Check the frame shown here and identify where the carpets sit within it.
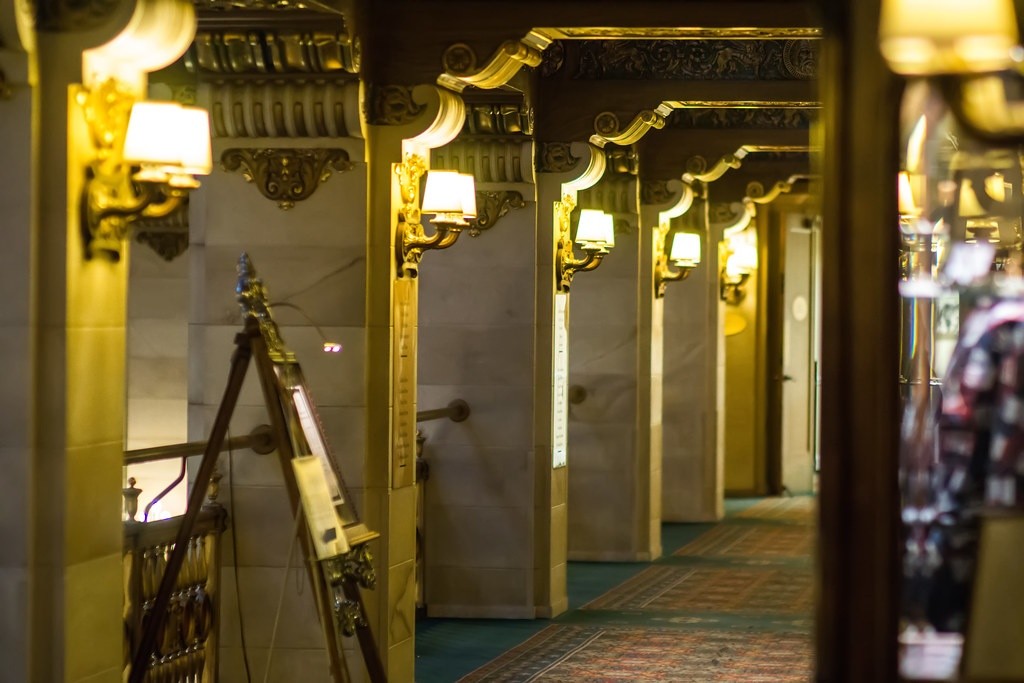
[459,494,814,683]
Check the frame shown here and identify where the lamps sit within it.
[654,232,701,298]
[83,98,213,263]
[556,208,615,292]
[720,246,758,300]
[396,168,478,277]
